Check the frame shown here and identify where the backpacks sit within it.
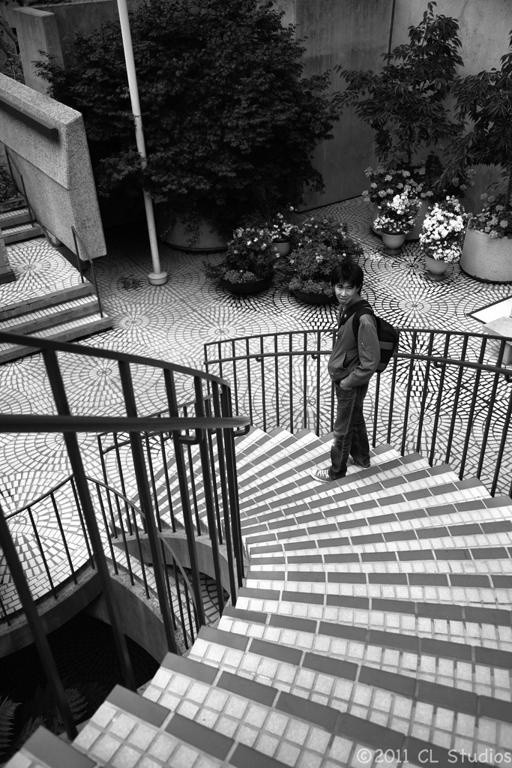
[353,308,397,373]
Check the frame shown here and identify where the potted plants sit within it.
[30,0,340,257]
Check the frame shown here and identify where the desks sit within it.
[465,293,512,372]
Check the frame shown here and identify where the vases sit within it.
[421,253,449,282]
[460,227,512,283]
[381,229,406,255]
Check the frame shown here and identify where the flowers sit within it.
[362,163,423,236]
[453,164,512,241]
[418,195,467,262]
[201,204,367,308]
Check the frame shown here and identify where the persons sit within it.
[310,261,380,483]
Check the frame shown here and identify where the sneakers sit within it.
[348,454,371,469]
[309,469,333,483]
